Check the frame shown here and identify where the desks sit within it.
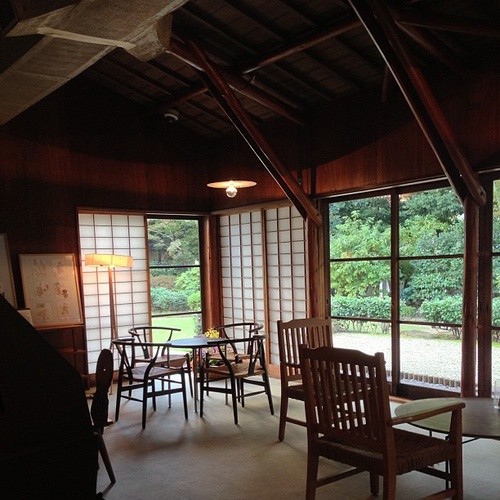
[165,336,230,400]
[395,396,500,444]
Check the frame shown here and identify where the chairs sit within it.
[90,349,117,483]
[111,338,188,430]
[298,344,467,500]
[198,333,274,424]
[127,326,194,397]
[276,315,371,442]
[202,322,264,402]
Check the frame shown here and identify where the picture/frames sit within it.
[17,252,85,331]
[1,232,17,310]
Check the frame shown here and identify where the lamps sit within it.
[85,254,135,395]
[205,129,258,199]
